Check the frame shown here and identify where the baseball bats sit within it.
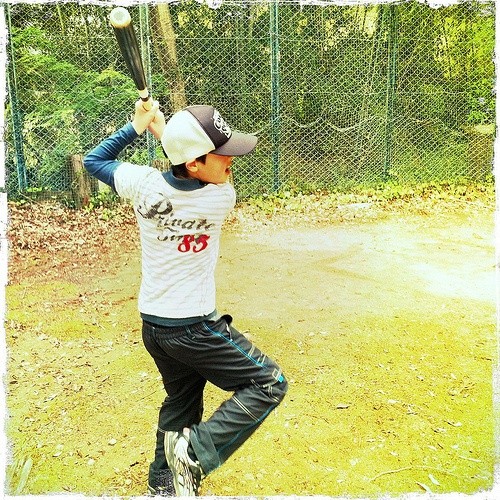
[110,8,153,111]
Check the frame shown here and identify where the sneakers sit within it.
[148,484,175,496]
[164,427,207,497]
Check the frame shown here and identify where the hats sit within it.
[160,105,258,166]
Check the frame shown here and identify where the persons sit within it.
[83,97,288,497]
